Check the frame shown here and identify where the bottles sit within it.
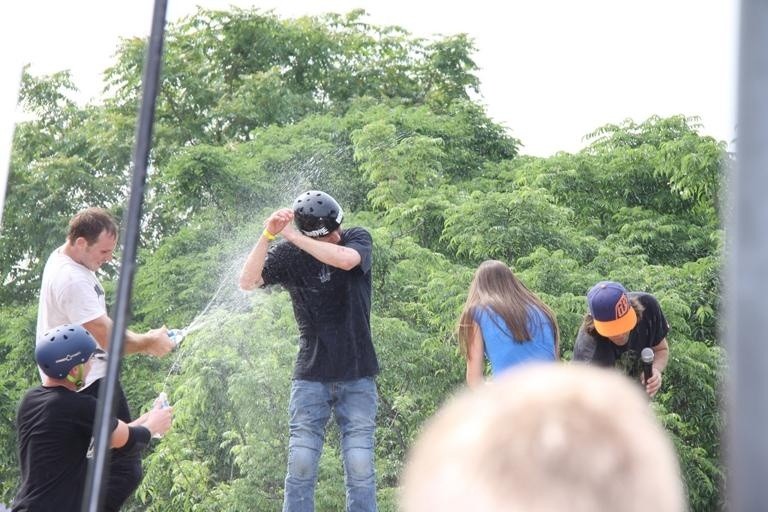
[168,326,190,345]
[150,389,171,438]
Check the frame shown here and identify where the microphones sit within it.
[641,347,654,384]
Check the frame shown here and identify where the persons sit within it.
[569,280,672,400]
[393,361,688,512]
[11,324,176,512]
[240,188,379,511]
[455,258,560,388]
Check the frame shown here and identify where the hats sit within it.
[589,280,640,339]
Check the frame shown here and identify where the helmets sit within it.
[293,190,346,238]
[33,323,104,381]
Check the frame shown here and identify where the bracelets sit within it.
[29,207,176,423]
[126,424,152,453]
[262,226,281,242]
